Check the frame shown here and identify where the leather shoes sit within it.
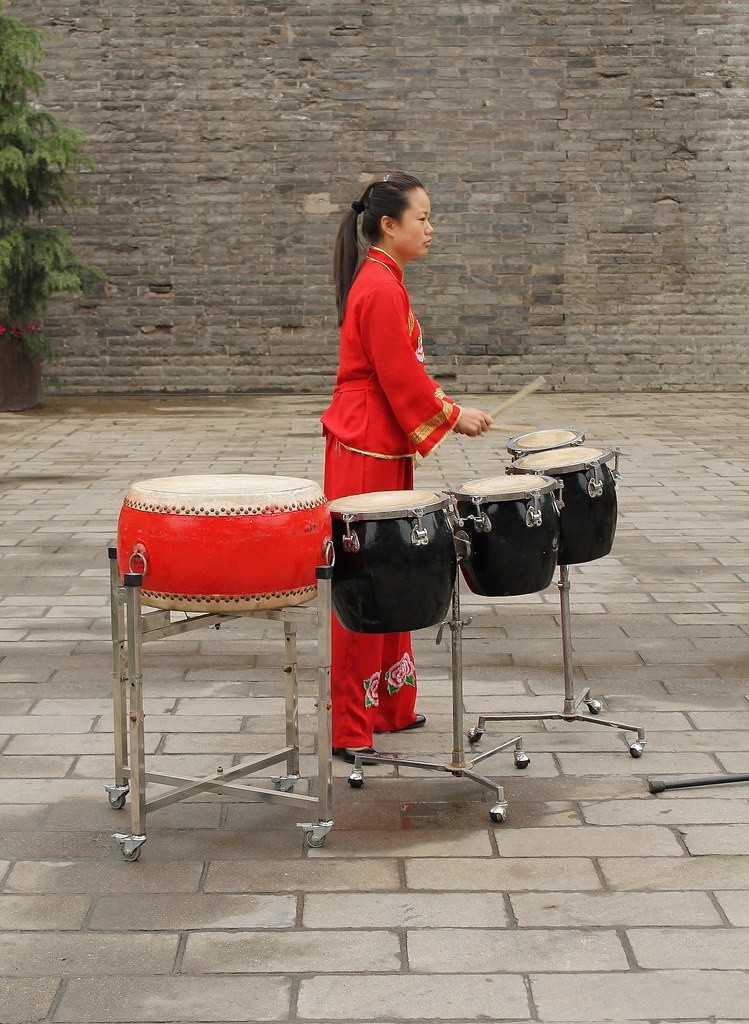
[408,714,427,730]
[331,745,380,765]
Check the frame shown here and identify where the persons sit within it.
[320,172,493,765]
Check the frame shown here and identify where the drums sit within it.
[450,473,560,598]
[328,488,458,635]
[506,426,585,464]
[508,442,617,566]
[116,472,334,614]
